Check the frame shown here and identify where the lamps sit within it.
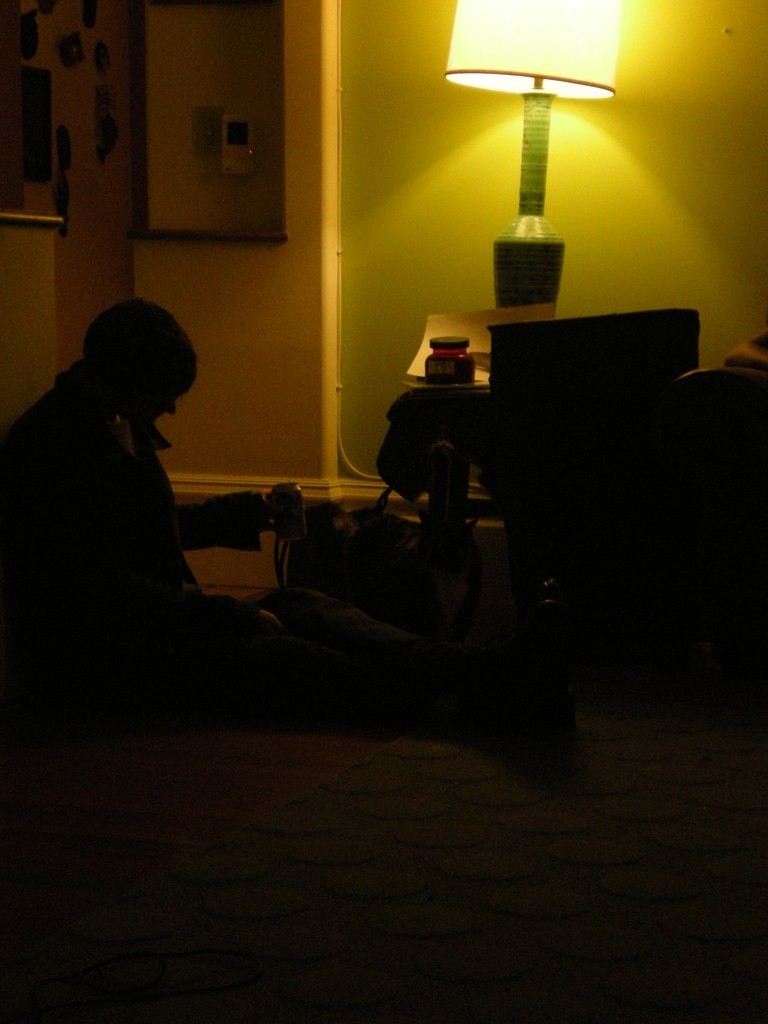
[444,1,623,305]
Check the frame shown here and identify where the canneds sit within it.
[424,337,475,386]
[271,482,307,540]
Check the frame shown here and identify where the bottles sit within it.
[425,337,474,383]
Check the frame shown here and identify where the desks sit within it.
[379,388,494,541]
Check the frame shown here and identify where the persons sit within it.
[1,300,579,751]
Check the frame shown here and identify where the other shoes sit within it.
[509,601,578,750]
